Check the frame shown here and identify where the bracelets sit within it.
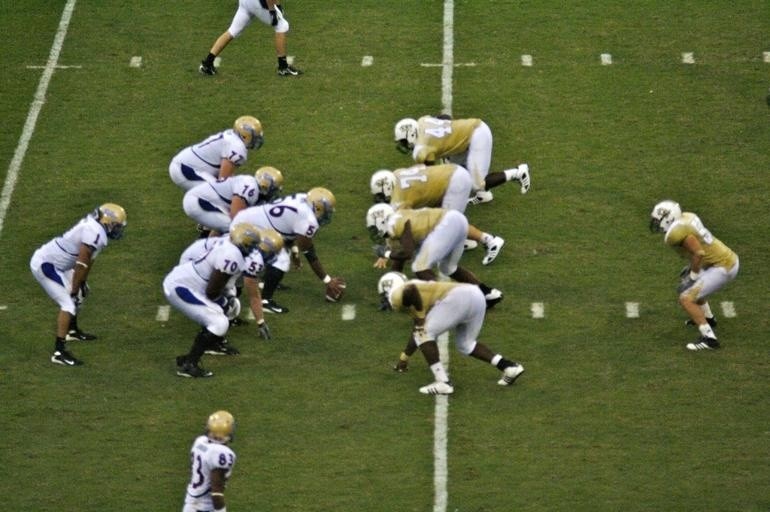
[385,250,392,259]
[399,352,410,363]
[689,268,700,281]
[214,505,226,512]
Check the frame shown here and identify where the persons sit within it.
[179,409,237,512]
[170,115,263,236]
[370,163,506,266]
[649,199,740,349]
[374,270,525,394]
[30,202,128,365]
[182,167,283,238]
[163,223,284,376]
[229,187,346,314]
[392,114,530,194]
[366,203,502,310]
[197,0,304,76]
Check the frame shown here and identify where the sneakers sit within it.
[199,64,217,75]
[685,315,718,327]
[485,288,504,309]
[65,330,96,342]
[482,236,505,265]
[686,337,720,351]
[258,282,289,314]
[176,317,250,378]
[467,190,493,205]
[51,351,84,366]
[515,163,531,194]
[464,239,479,250]
[497,363,525,385]
[277,65,303,76]
[418,382,456,395]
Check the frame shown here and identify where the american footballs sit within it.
[326,277,346,302]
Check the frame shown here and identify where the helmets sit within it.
[378,271,408,311]
[230,223,284,260]
[304,187,337,226]
[365,202,395,246]
[94,203,128,240]
[207,410,235,443]
[254,166,284,195]
[394,118,420,154]
[370,169,397,203]
[234,115,264,151]
[650,200,682,234]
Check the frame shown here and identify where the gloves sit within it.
[270,5,282,26]
[71,280,88,306]
[677,266,696,294]
[257,323,273,340]
